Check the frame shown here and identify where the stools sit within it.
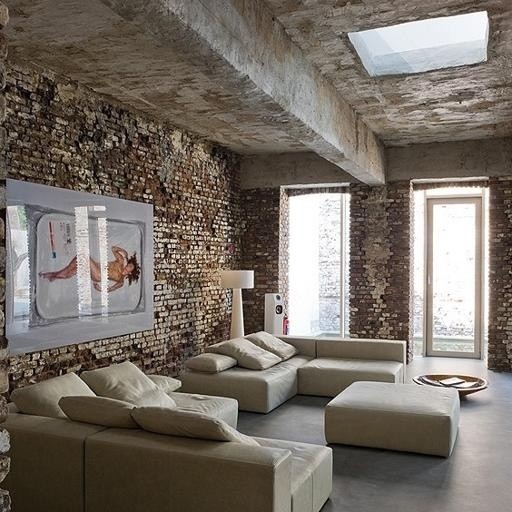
[324,381,460,456]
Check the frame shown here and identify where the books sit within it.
[438,376,464,387]
[421,376,443,386]
[451,381,478,388]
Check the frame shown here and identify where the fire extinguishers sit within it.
[283,314,289,335]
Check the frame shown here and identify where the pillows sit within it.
[79,361,177,410]
[146,375,182,395]
[10,372,98,419]
[132,406,262,448]
[183,353,237,373]
[217,337,282,370]
[244,330,300,362]
[58,396,141,430]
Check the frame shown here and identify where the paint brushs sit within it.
[49,221,56,258]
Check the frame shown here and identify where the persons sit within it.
[37,243,142,294]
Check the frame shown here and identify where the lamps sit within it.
[221,270,254,340]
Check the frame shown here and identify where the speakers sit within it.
[264,292,284,335]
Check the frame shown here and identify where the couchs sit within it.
[182,335,407,414]
[0,374,333,512]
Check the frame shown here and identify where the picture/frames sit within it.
[5,178,155,357]
